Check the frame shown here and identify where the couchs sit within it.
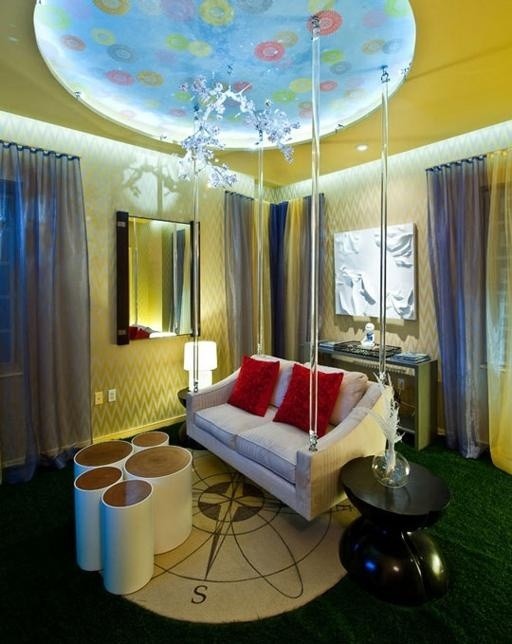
[187,352,392,521]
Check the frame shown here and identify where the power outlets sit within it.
[108,389,116,402]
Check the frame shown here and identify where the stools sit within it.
[67,429,193,594]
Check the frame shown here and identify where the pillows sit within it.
[226,353,344,436]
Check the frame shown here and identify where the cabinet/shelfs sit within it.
[329,350,438,451]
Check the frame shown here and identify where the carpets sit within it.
[99,449,359,625]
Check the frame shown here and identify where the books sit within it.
[388,351,430,364]
[319,341,338,351]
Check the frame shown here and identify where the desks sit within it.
[337,453,451,606]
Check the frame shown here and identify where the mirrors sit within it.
[124,212,194,343]
